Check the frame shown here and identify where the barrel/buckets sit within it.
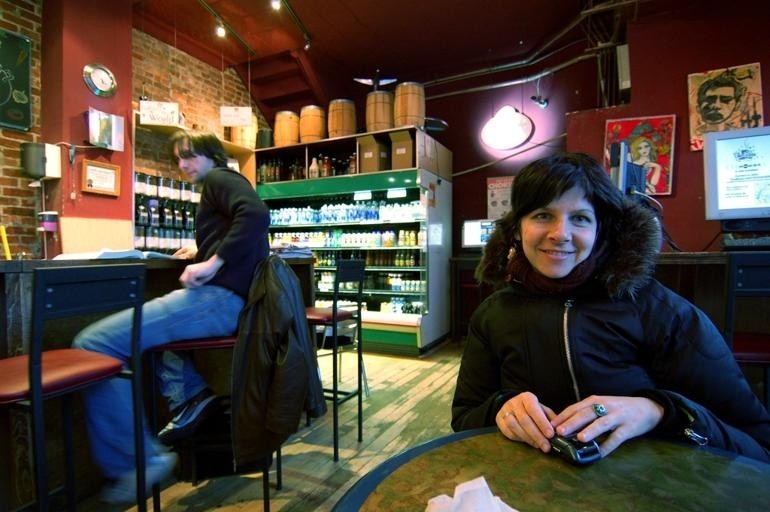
[231,113,259,148]
[274,81,425,148]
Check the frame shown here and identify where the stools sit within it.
[731,330,769,408]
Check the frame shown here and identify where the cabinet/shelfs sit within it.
[131,108,257,252]
[253,125,454,360]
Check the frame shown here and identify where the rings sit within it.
[590,401,608,417]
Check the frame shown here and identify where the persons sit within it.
[604,122,670,193]
[67,126,270,507]
[450,150,768,466]
[694,71,751,131]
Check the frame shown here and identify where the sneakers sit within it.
[157,387,219,441]
[100,452,179,505]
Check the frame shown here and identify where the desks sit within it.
[725,250,770,351]
[652,250,732,338]
[327,424,770,511]
[450,253,488,341]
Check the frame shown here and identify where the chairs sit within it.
[149,255,283,511]
[305,257,366,463]
[0,262,147,512]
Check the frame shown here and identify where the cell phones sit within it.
[550,433,601,465]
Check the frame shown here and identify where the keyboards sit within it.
[721,237,770,250]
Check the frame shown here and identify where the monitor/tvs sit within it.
[609,141,647,204]
[460,218,500,251]
[703,124,769,220]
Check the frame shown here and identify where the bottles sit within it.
[256,151,357,183]
[136,171,199,255]
[268,201,426,315]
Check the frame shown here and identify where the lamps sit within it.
[480,86,534,151]
